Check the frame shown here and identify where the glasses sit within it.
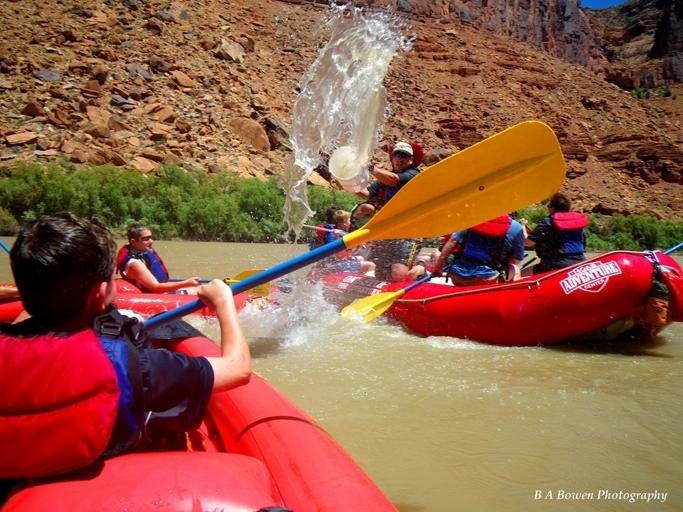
[546,204,554,209]
[139,236,153,241]
[395,152,410,158]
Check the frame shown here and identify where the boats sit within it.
[1,312,402,510]
[305,244,683,355]
[0,280,274,321]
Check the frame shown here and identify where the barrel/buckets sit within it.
[327,145,370,193]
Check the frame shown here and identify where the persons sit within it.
[326,140,421,279]
[426,210,529,288]
[117,224,201,293]
[518,191,588,275]
[308,204,450,301]
[0,209,253,496]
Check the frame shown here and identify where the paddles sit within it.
[340,266,451,322]
[166,269,269,293]
[143,120,563,329]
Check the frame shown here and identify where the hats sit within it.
[393,142,414,156]
